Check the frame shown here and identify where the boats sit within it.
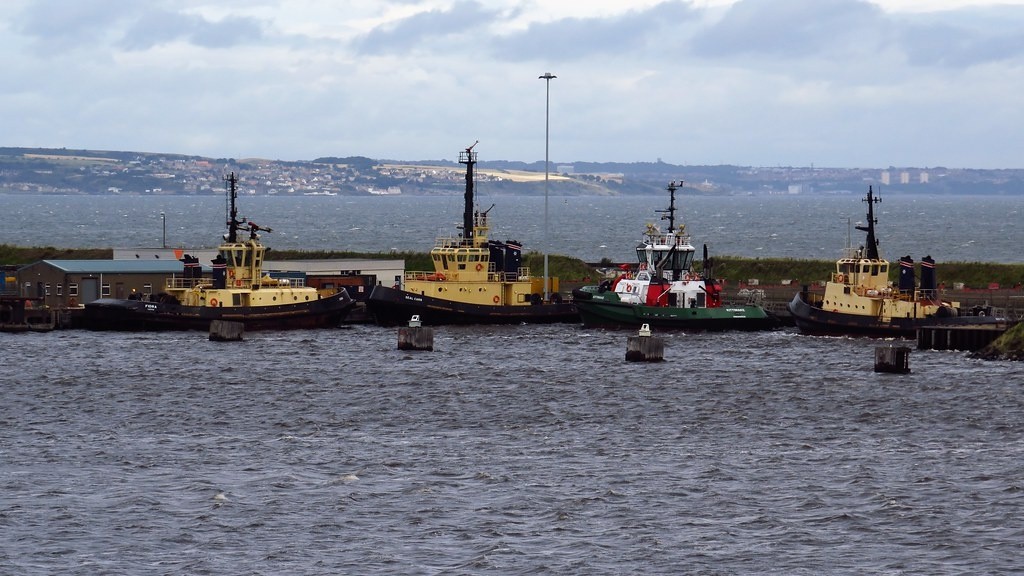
[786,182,1017,348]
[360,139,575,322]
[88,171,360,331]
[570,176,777,332]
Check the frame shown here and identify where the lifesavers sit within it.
[837,275,843,282]
[979,311,986,316]
[627,285,631,292]
[493,296,500,303]
[476,264,482,271]
[210,298,218,306]
[685,273,691,280]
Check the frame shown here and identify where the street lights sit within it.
[160,211,166,249]
[537,73,557,306]
